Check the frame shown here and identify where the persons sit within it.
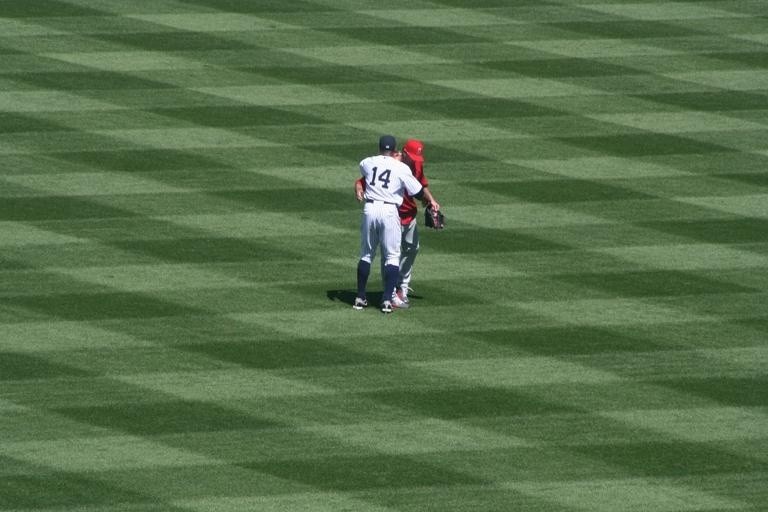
[353,136,440,313]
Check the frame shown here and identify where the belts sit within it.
[365,198,396,205]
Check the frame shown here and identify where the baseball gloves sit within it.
[424,204,444,229]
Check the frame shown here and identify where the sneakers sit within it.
[352,295,368,310]
[380,285,414,312]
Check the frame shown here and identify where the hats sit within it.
[403,140,425,162]
[379,135,396,151]
